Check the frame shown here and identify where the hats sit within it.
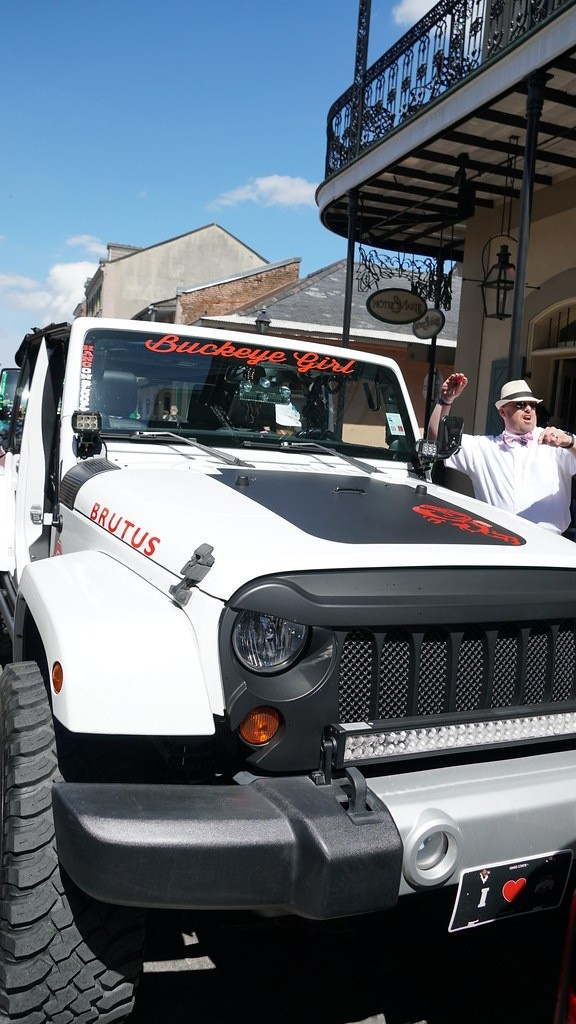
[494,379,543,411]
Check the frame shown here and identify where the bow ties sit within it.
[502,426,536,447]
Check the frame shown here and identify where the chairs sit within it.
[93,362,307,437]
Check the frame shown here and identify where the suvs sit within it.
[0,312,576,1024]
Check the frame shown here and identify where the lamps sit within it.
[476,134,520,322]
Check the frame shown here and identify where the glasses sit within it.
[504,401,537,409]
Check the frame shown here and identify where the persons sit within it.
[162,405,185,422]
[426,373,575,541]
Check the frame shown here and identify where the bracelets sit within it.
[436,397,454,406]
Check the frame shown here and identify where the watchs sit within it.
[559,431,575,449]
